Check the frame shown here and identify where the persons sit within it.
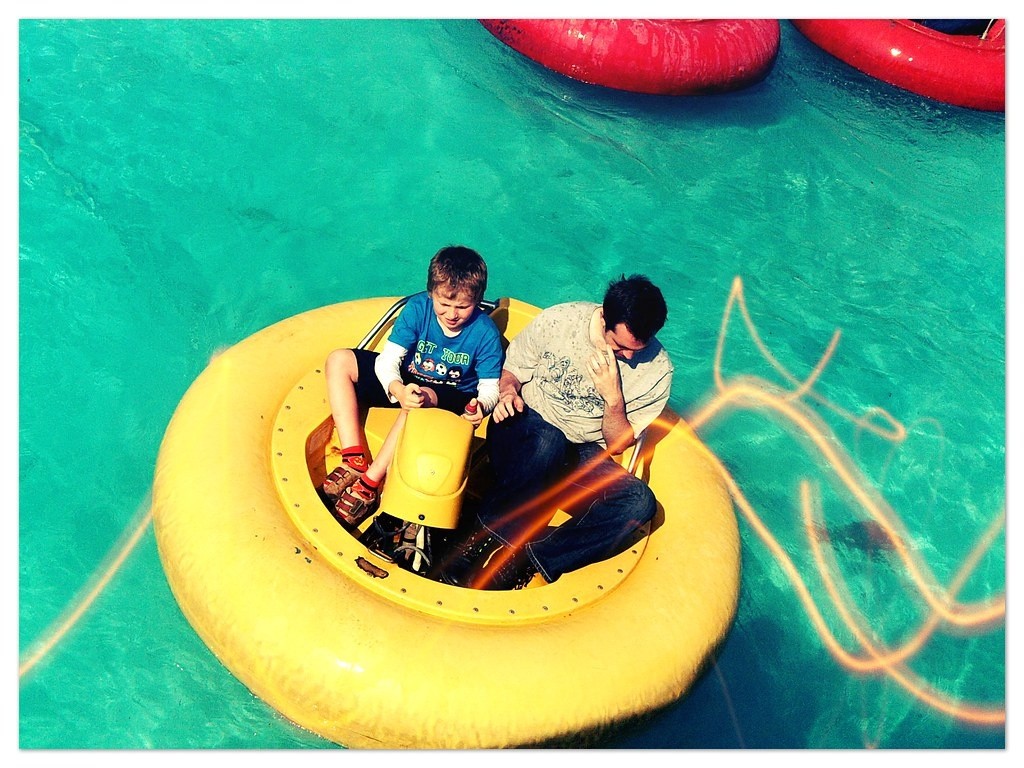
[316,246,504,528]
[435,273,675,592]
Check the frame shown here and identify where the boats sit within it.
[477,18,781,93]
[793,19,1005,113]
[151,294,740,751]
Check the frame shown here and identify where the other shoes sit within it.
[466,543,537,591]
[434,516,500,587]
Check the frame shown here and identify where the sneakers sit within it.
[330,478,377,531]
[316,464,366,506]
